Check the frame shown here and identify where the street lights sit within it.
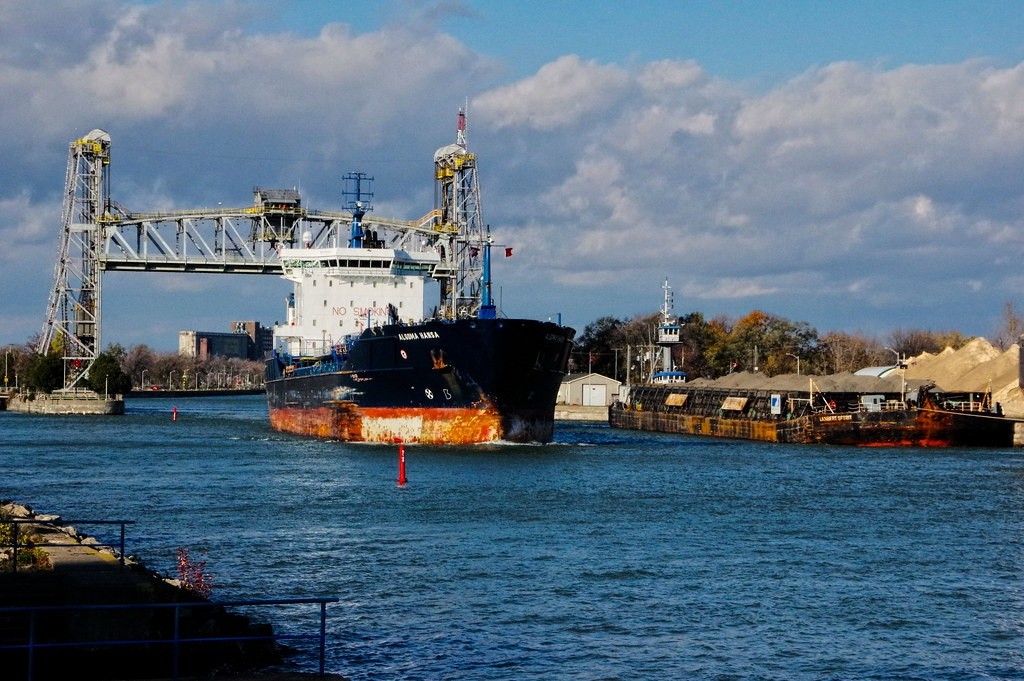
[195,372,202,390]
[785,352,800,375]
[4,351,9,392]
[141,369,148,390]
[207,372,258,389]
[105,374,109,400]
[169,369,176,390]
[884,347,899,368]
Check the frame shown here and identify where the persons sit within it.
[828,398,836,413]
[346,336,353,351]
[278,346,281,356]
[330,346,338,361]
[837,399,848,413]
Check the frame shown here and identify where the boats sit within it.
[259,163,576,452]
[608,381,1014,450]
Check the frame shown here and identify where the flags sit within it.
[730,363,740,369]
[505,248,512,258]
[50,326,63,352]
[590,356,598,362]
